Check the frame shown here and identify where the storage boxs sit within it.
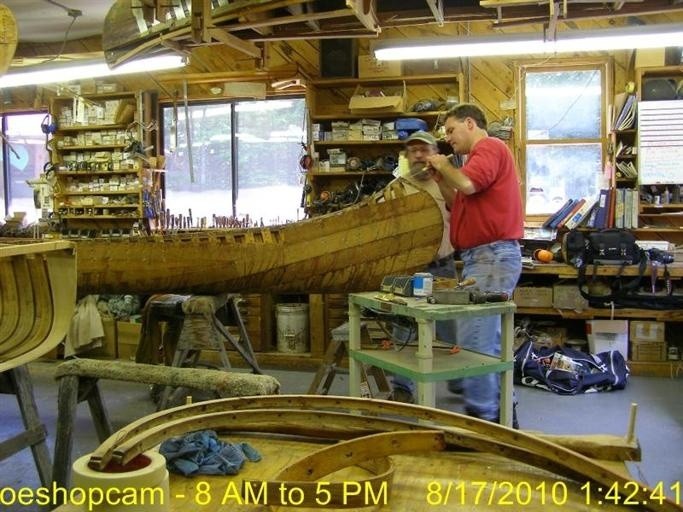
[358,55,402,76]
[513,279,668,361]
[58,316,142,360]
[348,96,403,113]
[634,47,665,68]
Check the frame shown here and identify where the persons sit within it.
[426,103,524,429]
[374,131,463,406]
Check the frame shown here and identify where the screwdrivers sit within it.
[453,276,477,289]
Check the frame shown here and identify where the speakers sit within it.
[319,38,359,79]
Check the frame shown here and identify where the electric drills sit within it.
[469,287,508,304]
[532,248,562,264]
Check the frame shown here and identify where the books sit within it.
[542,187,639,233]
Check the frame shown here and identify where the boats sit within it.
[1,175,445,296]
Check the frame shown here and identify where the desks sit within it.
[51,436,648,512]
[349,291,517,430]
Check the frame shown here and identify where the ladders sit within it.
[306,322,395,401]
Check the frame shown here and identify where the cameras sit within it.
[649,247,674,264]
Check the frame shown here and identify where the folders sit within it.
[594,189,609,230]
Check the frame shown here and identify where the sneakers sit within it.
[389,388,414,404]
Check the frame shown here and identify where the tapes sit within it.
[71,450,169,512]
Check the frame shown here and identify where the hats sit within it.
[407,131,436,145]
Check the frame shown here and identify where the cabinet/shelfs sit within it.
[610,65,683,232]
[50,88,144,236]
[512,264,683,378]
[224,293,271,351]
[306,72,466,357]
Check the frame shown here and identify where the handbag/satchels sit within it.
[562,228,641,265]
[514,339,629,395]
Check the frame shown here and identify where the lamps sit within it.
[0,54,191,88]
[374,3,683,60]
